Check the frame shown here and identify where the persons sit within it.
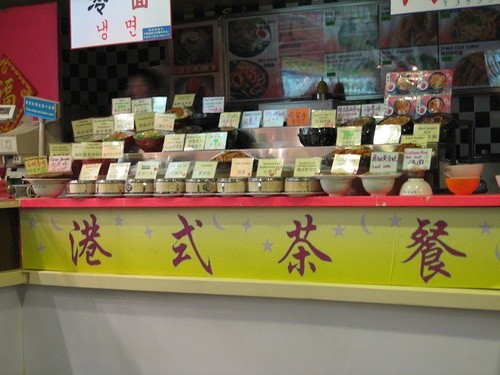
[129,68,164,99]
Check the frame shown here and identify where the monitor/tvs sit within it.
[1,118,61,157]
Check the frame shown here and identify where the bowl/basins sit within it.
[320,178,354,195]
[228,19,272,57]
[166,107,194,127]
[444,164,484,195]
[75,129,168,151]
[400,177,434,195]
[385,81,427,115]
[28,181,65,199]
[72,158,118,175]
[231,59,270,98]
[495,175,500,189]
[297,113,459,146]
[361,178,395,196]
[393,73,447,114]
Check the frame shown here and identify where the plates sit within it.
[314,175,357,179]
[21,179,72,180]
[358,173,402,178]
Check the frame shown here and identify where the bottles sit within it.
[335,77,344,94]
[317,77,328,94]
